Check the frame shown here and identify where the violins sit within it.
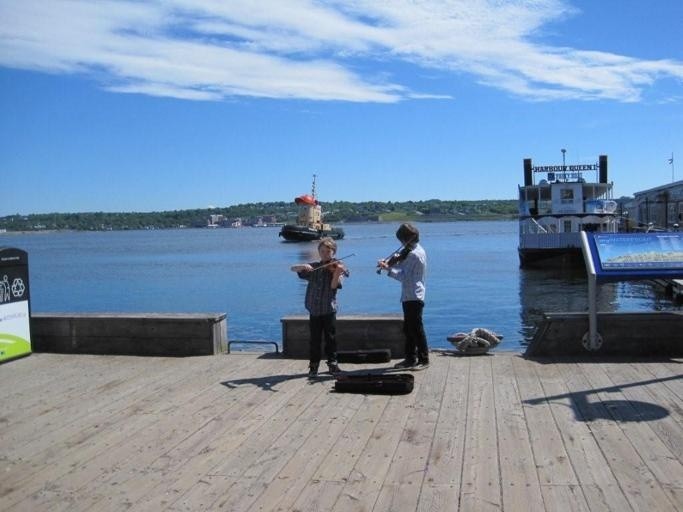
[377,247,410,268]
[321,258,349,277]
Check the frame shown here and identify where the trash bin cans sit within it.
[0,244,32,365]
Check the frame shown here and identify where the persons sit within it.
[314,220,320,233]
[377,222,430,372]
[319,220,323,233]
[288,236,347,378]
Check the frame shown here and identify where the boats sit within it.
[278,172,346,242]
[515,146,620,268]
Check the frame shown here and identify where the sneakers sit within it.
[325,360,341,375]
[413,361,429,371]
[395,360,414,369]
[308,362,319,377]
